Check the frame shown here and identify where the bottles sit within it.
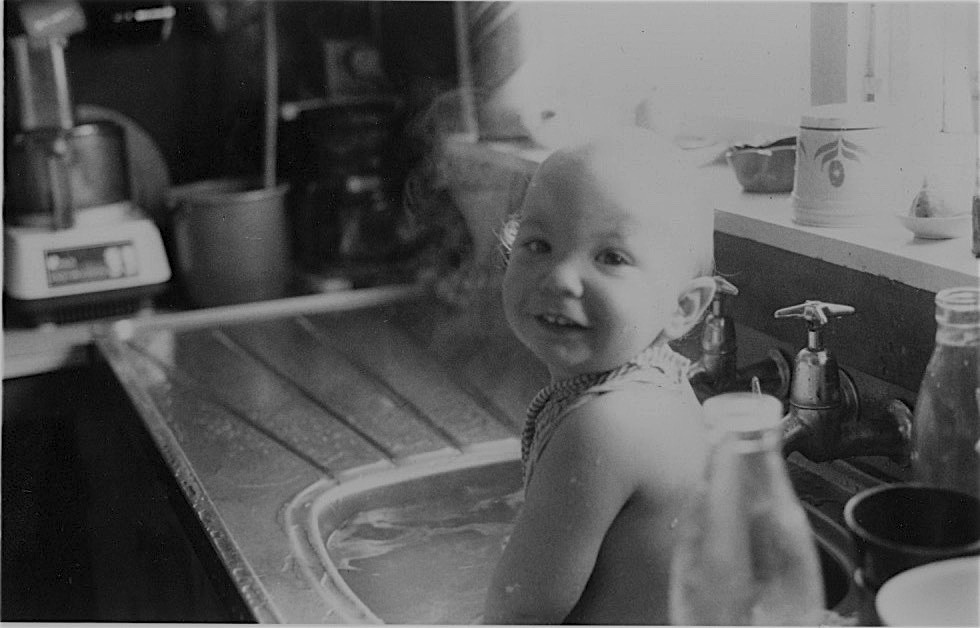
[910,285,980,495]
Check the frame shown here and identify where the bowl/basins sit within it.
[726,145,797,192]
[895,212,972,240]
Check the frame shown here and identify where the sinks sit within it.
[279,438,856,626]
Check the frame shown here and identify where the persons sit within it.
[484,128,738,626]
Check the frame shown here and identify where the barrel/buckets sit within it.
[166,178,292,306]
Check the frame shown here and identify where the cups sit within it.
[875,555,980,628]
[844,479,980,628]
[790,102,896,229]
[703,391,784,515]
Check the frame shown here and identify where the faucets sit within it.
[750,300,916,462]
[683,273,790,408]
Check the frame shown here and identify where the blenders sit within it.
[2,2,185,315]
